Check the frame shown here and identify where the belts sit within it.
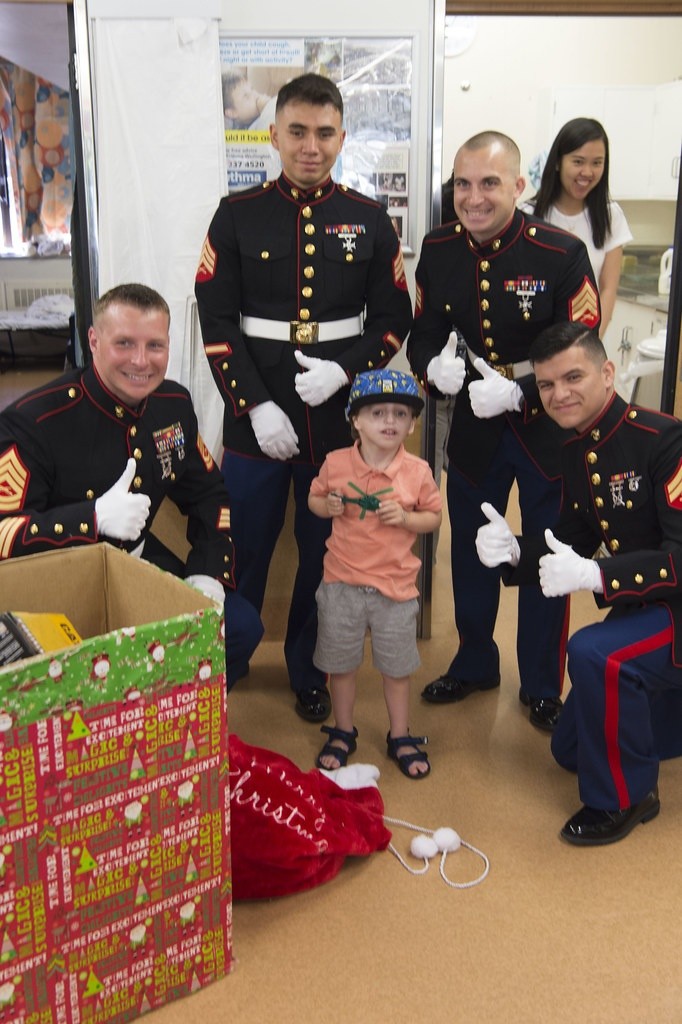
[465,344,533,382]
[237,304,368,345]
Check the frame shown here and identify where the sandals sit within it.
[386,725,431,780]
[315,723,357,771]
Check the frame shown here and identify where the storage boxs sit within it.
[1,539,236,1024]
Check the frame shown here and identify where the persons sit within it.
[303,368,445,780]
[222,74,270,129]
[0,283,271,692]
[475,322,682,847]
[521,117,633,349]
[193,73,414,725]
[404,130,607,735]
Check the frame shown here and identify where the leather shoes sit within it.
[518,685,564,732]
[294,685,332,722]
[421,674,502,704]
[560,781,660,846]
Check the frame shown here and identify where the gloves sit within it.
[538,529,604,598]
[185,574,224,616]
[473,500,522,568]
[427,331,466,396]
[468,358,522,418]
[250,400,300,460]
[95,458,150,541]
[293,348,350,407]
